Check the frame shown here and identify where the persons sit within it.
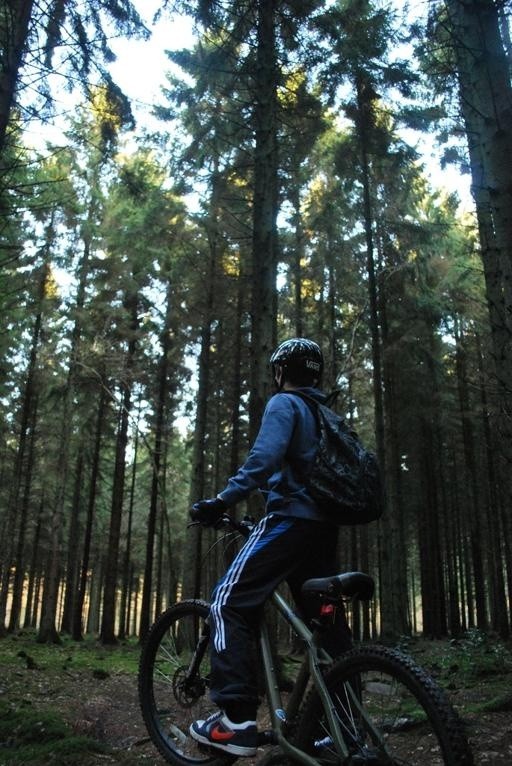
[189,338,362,759]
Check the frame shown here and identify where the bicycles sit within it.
[136,512,474,766]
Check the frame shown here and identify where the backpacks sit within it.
[278,390,385,525]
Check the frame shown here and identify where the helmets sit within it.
[270,337,322,372]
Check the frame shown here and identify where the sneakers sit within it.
[314,729,362,749]
[190,710,257,757]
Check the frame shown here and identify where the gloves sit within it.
[188,498,225,522]
